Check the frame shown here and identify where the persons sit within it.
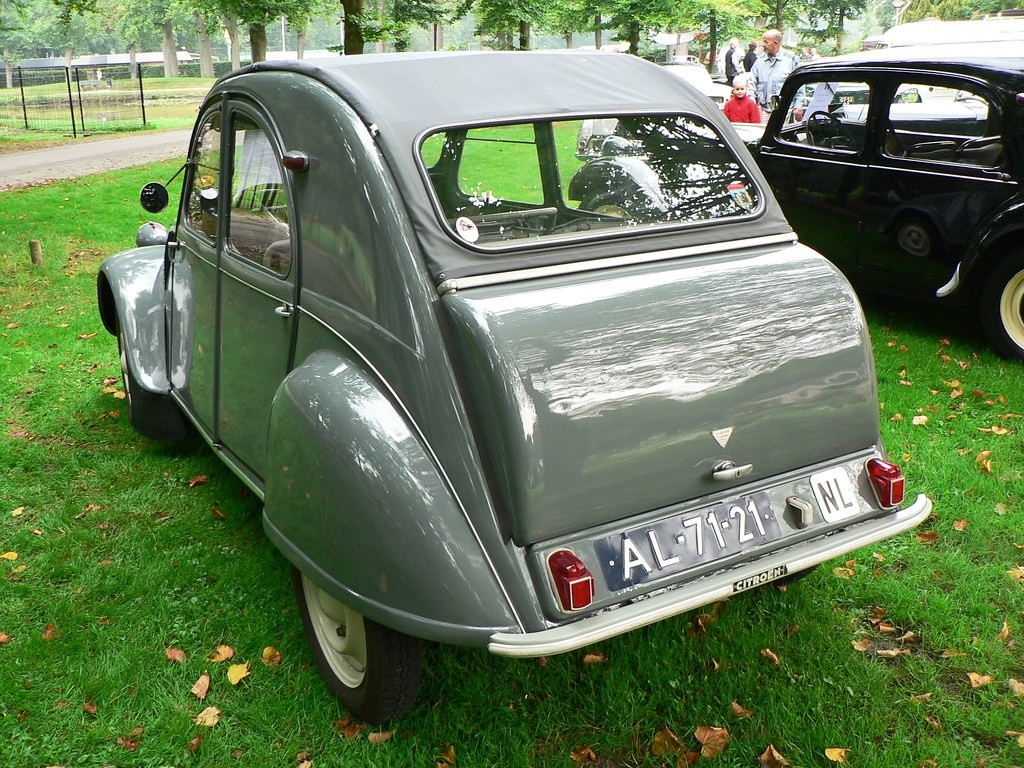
[748,30,806,125]
[723,76,761,124]
[706,38,820,86]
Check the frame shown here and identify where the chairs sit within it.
[954,133,1004,166]
[902,142,958,159]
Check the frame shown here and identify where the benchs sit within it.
[264,204,560,277]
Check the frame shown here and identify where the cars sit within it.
[569,5,1024,369]
[97,46,934,730]
